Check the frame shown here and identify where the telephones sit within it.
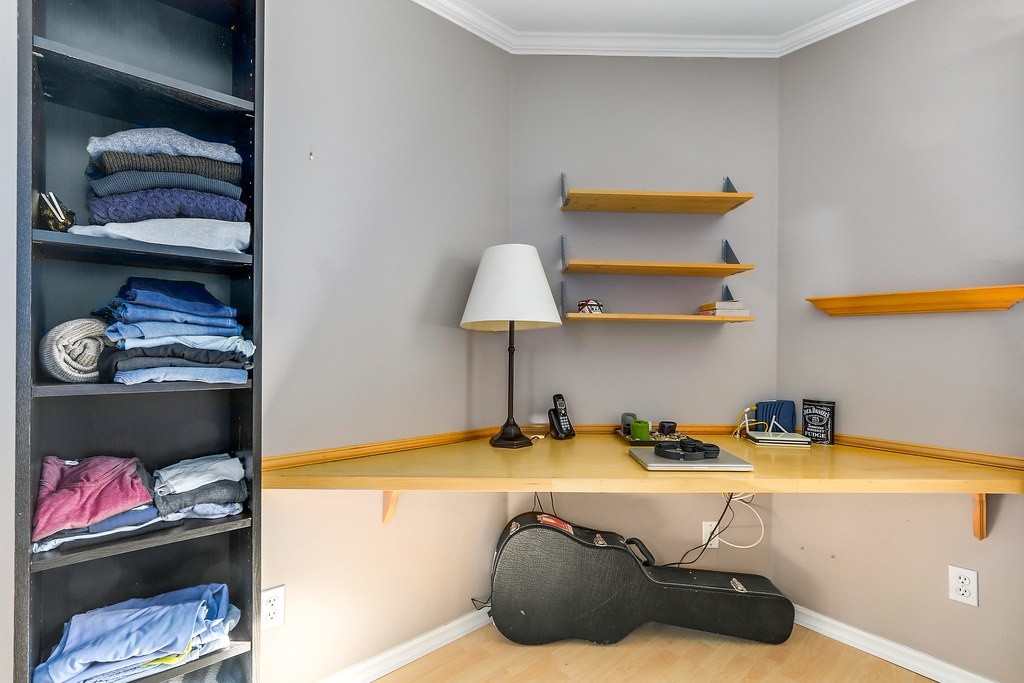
[548,394,576,440]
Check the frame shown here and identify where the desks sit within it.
[265,424,1024,541]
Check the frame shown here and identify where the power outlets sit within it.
[703,521,720,549]
[261,584,285,632]
[947,565,980,607]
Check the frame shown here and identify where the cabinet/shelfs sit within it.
[559,171,756,323]
[0,0,267,683]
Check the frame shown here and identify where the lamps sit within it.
[459,243,564,448]
